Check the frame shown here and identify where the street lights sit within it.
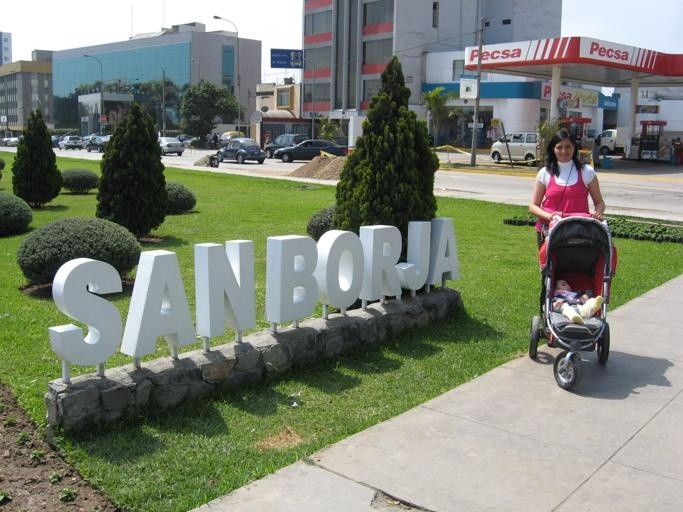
[213,14,240,137]
[192,58,200,79]
[83,53,105,136]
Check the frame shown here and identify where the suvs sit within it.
[262,134,309,159]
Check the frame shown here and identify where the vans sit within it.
[220,131,244,140]
[489,132,543,166]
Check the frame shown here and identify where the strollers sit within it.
[528,213,616,390]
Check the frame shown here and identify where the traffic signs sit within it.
[270,48,302,68]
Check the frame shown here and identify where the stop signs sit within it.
[100,115,106,123]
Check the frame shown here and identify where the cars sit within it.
[272,139,347,162]
[0,134,184,156]
[216,137,265,163]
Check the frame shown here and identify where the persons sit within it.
[549,278,604,325]
[671,137,682,165]
[527,126,606,319]
[212,132,221,148]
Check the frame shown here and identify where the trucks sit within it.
[597,127,617,157]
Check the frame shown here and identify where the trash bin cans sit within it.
[428,133,434,147]
